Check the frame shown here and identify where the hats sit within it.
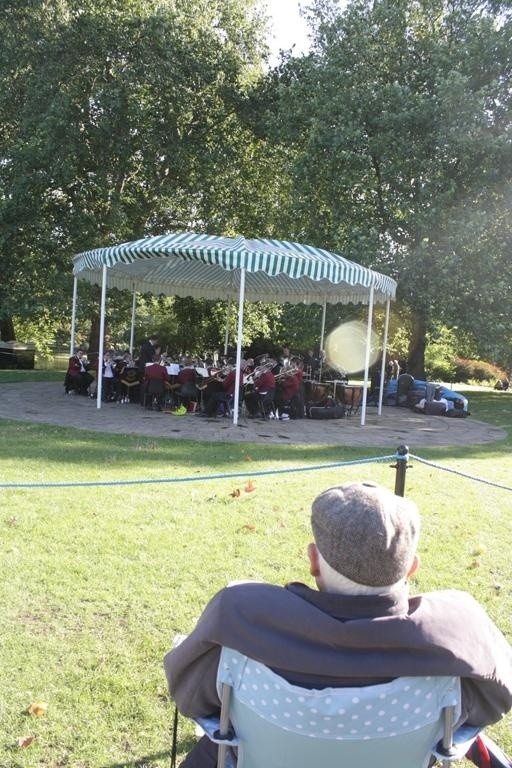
[311,481,419,585]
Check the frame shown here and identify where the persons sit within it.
[163,481,512,768]
[393,359,399,379]
[494,380,503,390]
[93,335,350,417]
[68,349,95,396]
[387,360,394,382]
[503,378,509,391]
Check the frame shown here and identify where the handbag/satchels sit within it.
[305,394,346,419]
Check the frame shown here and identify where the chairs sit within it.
[395,375,415,407]
[61,350,370,426]
[166,632,475,768]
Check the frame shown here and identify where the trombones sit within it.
[275,368,298,382]
[196,365,235,391]
[243,361,276,388]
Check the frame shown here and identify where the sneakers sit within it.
[67,387,293,422]
